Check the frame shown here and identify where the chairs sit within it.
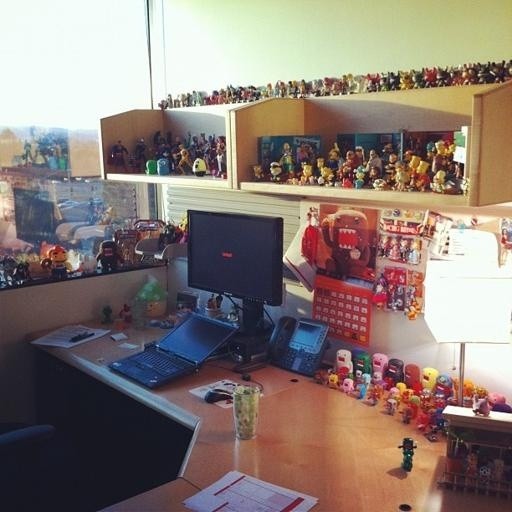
[1,423,57,512]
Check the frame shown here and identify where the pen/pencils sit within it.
[69,332,94,343]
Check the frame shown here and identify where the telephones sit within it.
[266,316,330,377]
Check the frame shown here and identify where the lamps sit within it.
[423,261,512,406]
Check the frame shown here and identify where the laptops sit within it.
[107,311,240,390]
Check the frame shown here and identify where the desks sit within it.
[31,315,512,512]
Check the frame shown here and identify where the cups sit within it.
[231,384,263,440]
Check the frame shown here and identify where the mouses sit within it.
[205,389,234,403]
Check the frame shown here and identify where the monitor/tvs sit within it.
[14,188,54,252]
[187,210,283,336]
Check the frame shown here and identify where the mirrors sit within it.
[0,0,169,292]
